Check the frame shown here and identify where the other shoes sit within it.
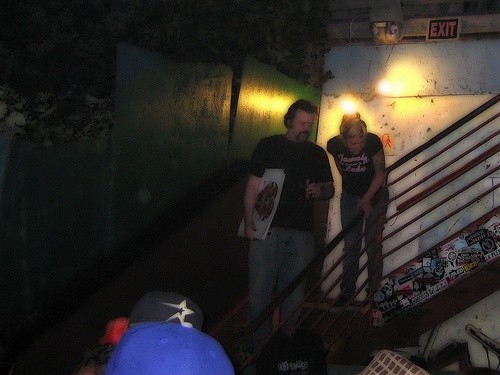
[333,295,354,307]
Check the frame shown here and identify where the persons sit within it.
[327,115,389,307]
[243,99,335,356]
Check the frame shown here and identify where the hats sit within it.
[128,290,204,331]
[100,317,236,375]
